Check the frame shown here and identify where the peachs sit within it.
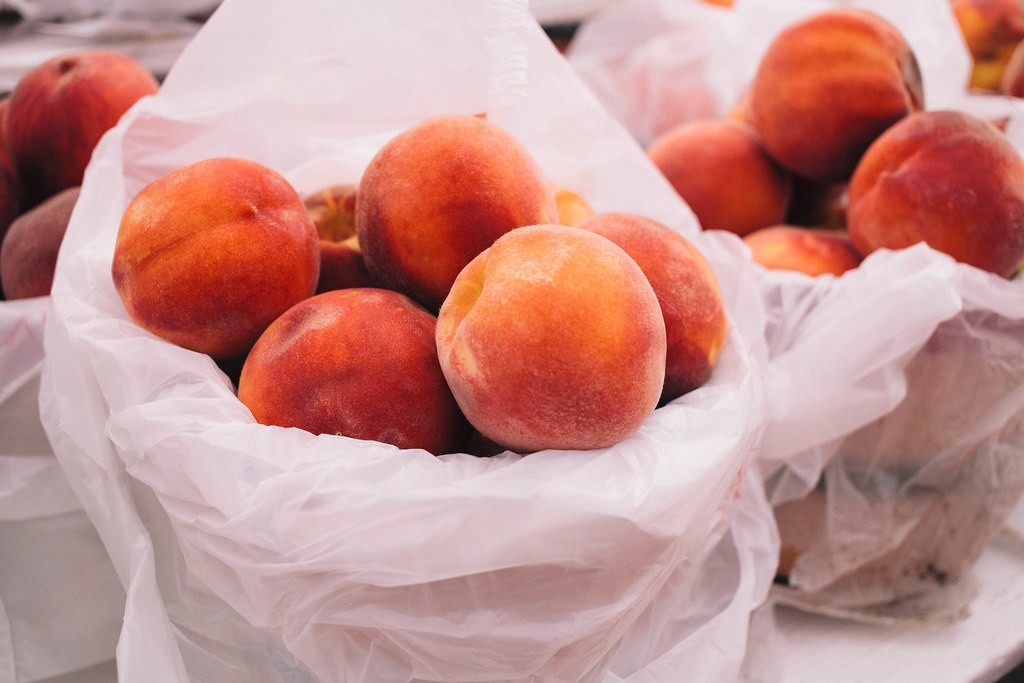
[0,1,1024,453]
